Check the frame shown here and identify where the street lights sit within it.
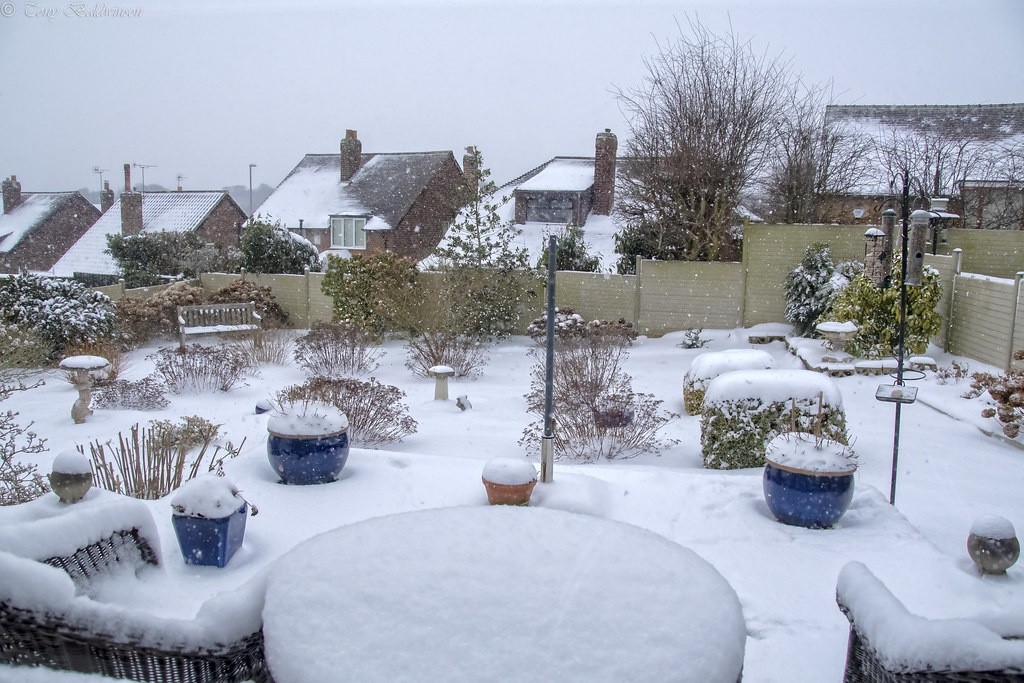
[249,163,258,222]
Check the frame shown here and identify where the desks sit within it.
[265,506,747,683]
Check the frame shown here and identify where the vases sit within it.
[482,477,538,504]
[763,453,857,530]
[256,406,267,414]
[172,503,248,569]
[267,429,351,483]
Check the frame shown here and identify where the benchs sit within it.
[834,561,1023,683]
[0,502,269,683]
[177,301,262,352]
